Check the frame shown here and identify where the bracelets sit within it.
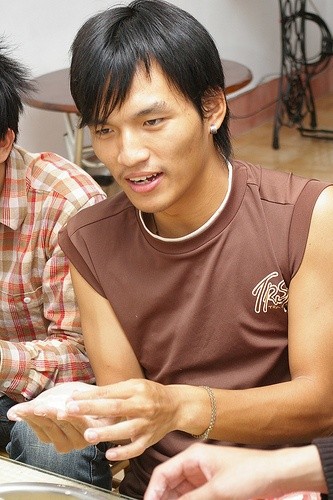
[193,386,218,440]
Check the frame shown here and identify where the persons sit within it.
[5,0,333,498]
[0,36,113,489]
[142,435,333,500]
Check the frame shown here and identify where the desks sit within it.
[18,59,253,177]
